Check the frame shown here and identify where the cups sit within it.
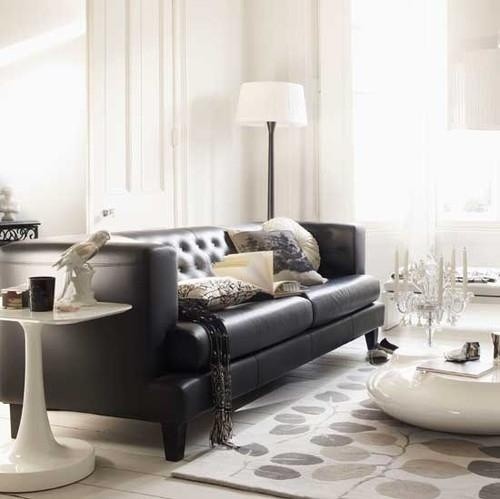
[29,277,55,312]
[490,331,500,363]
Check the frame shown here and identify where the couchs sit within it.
[385,267,500,297]
[0,222,385,462]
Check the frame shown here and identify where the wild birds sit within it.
[54,231,111,302]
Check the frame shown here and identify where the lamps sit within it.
[235,81,308,222]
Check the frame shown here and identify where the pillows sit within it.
[233,229,328,284]
[227,217,321,272]
[177,276,262,313]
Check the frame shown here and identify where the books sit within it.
[212,250,310,299]
[417,357,495,379]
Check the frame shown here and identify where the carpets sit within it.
[170,355,500,499]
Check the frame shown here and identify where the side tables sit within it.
[0,220,41,247]
[0,297,135,492]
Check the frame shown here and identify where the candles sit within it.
[462,247,468,296]
[403,248,408,287]
[450,248,456,296]
[393,249,399,292]
[438,251,444,307]
